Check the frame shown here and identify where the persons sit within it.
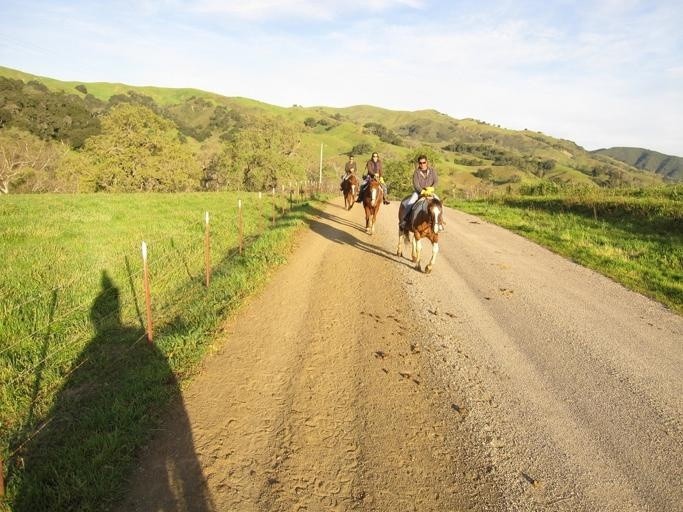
[355,152,390,204]
[399,155,437,231]
[340,156,358,191]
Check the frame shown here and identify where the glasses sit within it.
[418,161,427,164]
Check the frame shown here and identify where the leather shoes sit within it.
[355,197,362,203]
[383,200,390,205]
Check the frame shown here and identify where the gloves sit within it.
[420,187,434,197]
[373,174,383,184]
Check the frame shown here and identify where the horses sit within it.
[342,175,360,211]
[361,177,384,236]
[397,193,447,274]
